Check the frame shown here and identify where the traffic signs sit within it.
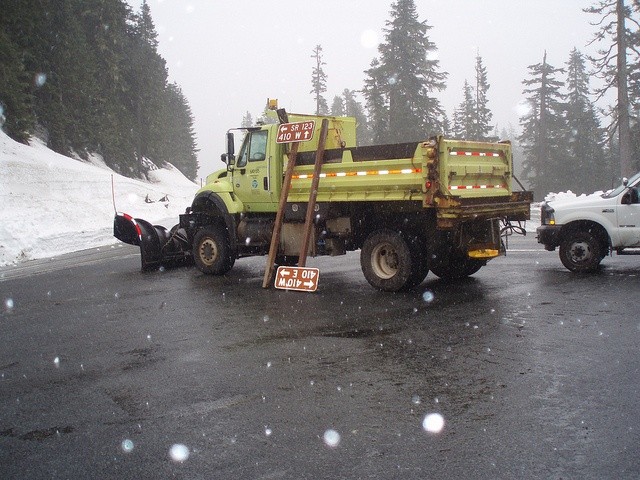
[274,266,319,292]
[276,120,315,144]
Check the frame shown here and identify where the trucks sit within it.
[179,100,534,290]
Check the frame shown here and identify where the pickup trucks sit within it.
[537,171,640,272]
